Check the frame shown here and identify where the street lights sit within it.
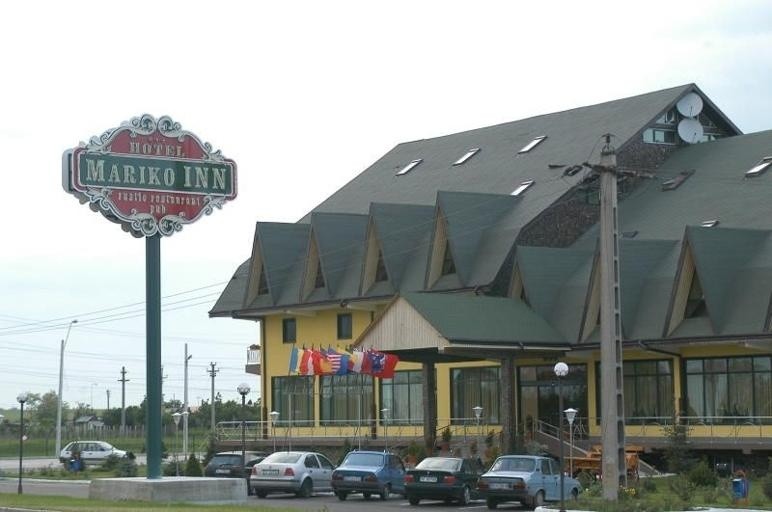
[237,383,251,471]
[171,411,181,451]
[269,410,280,451]
[474,406,483,459]
[564,408,578,480]
[554,361,570,511]
[16,392,28,494]
[380,408,390,450]
[55,320,77,458]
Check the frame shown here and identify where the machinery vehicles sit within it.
[564,443,646,489]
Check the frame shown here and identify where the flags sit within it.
[288,346,399,379]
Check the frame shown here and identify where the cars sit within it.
[331,450,407,500]
[251,450,338,497]
[478,455,584,509]
[405,457,486,505]
[60,440,127,466]
[204,450,268,494]
